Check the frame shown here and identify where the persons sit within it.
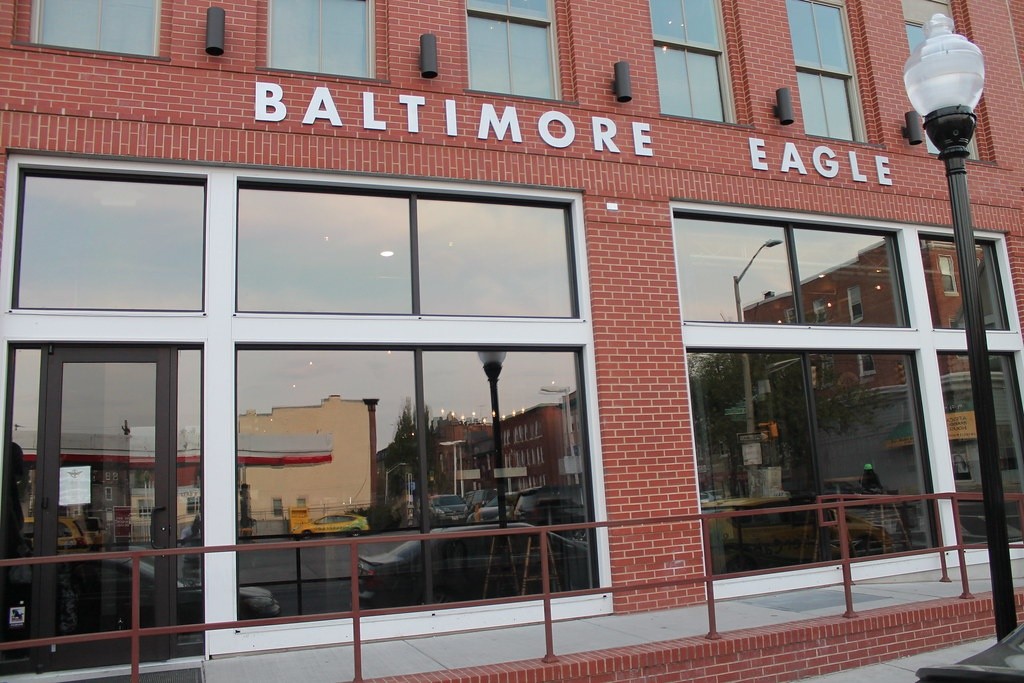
[179,514,202,586]
[859,464,883,493]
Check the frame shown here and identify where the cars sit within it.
[16,514,109,553]
[700,488,893,574]
[1,550,283,657]
[415,482,586,541]
[356,518,592,607]
[290,511,371,541]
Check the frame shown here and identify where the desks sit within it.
[701,496,791,573]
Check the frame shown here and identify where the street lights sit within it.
[385,462,409,504]
[476,351,515,595]
[440,440,467,495]
[898,10,1019,644]
[730,238,783,528]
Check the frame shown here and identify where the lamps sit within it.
[420,34,438,79]
[612,61,632,102]
[901,111,923,145]
[772,88,794,125]
[206,6,225,56]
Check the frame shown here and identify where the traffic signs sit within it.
[736,430,771,444]
